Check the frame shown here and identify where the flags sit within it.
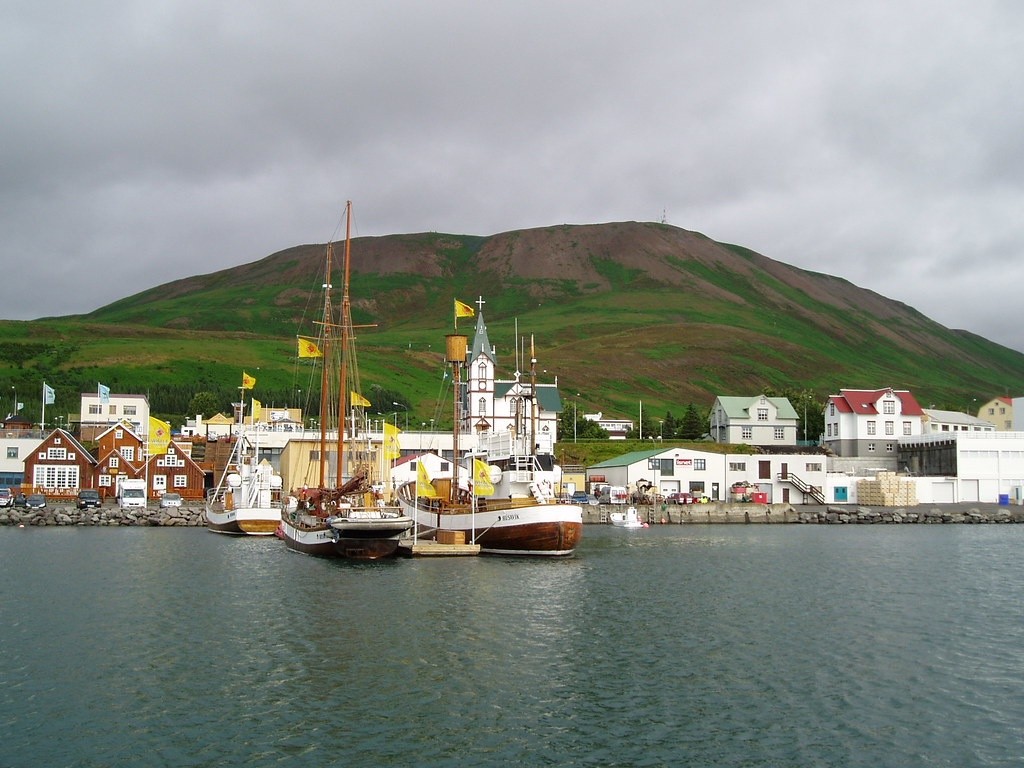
[455,300,474,317]
[417,461,437,498]
[149,416,170,454]
[17,403,24,411]
[45,384,54,404]
[383,423,402,461]
[253,400,261,419]
[297,338,323,357]
[474,458,494,496]
[242,372,256,389]
[99,385,110,404]
[351,391,371,407]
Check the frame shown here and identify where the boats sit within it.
[609,506,644,528]
[200,368,282,537]
[394,298,585,559]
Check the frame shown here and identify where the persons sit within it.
[20,492,27,508]
[375,490,385,512]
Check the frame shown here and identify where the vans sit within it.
[601,486,627,505]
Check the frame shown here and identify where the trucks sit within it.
[117,479,146,512]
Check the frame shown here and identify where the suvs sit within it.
[76,489,102,510]
[0,488,14,507]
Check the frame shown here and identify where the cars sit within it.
[664,493,694,504]
[15,493,46,510]
[572,491,589,504]
[159,493,184,508]
[587,494,599,507]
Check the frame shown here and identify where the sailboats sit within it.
[280,200,415,564]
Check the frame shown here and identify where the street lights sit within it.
[393,402,409,431]
[298,389,301,408]
[805,395,812,445]
[11,386,17,416]
[648,436,657,507]
[966,398,976,415]
[659,420,663,443]
[430,418,434,430]
